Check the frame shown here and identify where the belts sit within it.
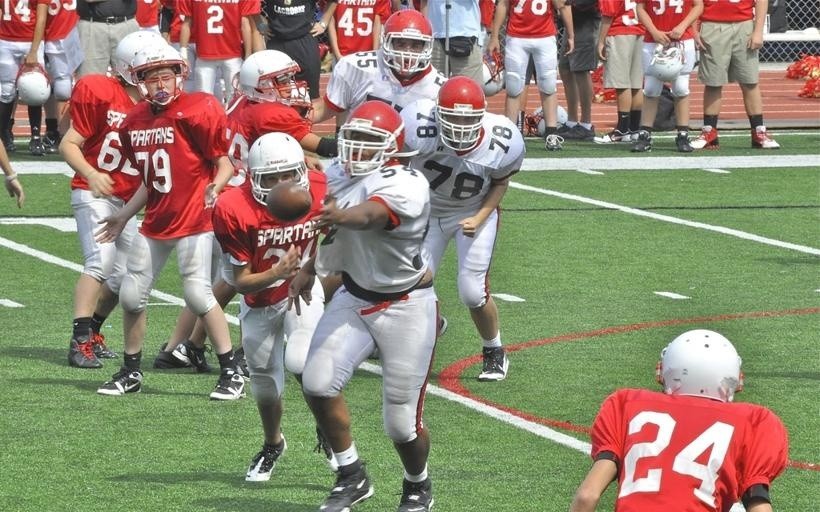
[80,16,134,24]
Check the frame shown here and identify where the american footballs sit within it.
[266,183,312,221]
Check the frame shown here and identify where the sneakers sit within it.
[319,462,374,512]
[153,339,251,377]
[69,339,102,369]
[676,125,720,153]
[4,136,16,151]
[751,126,781,149]
[398,478,434,511]
[210,368,246,400]
[90,334,122,357]
[28,131,59,155]
[546,121,652,153]
[96,366,144,396]
[478,346,510,382]
[244,432,286,481]
[315,427,340,473]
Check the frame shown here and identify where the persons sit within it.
[152,229,255,383]
[212,130,341,481]
[303,10,449,362]
[151,49,338,382]
[393,75,525,379]
[57,31,170,370]
[301,99,440,512]
[97,46,245,399]
[569,329,788,512]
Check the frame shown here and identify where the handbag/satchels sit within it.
[442,36,477,59]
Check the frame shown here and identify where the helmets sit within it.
[16,63,52,107]
[337,100,406,176]
[482,52,505,97]
[115,30,168,87]
[644,36,686,83]
[128,43,191,105]
[231,49,313,110]
[525,104,568,137]
[382,9,434,74]
[247,132,310,207]
[655,328,743,402]
[436,76,486,153]
